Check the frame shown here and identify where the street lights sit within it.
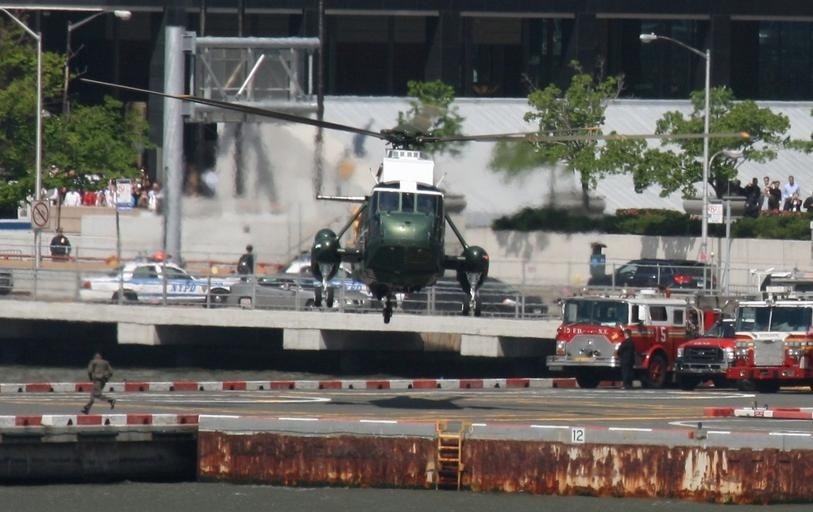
[640,33,711,260]
[64,10,130,115]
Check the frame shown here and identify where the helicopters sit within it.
[82,79,749,323]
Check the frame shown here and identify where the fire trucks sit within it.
[546,287,721,389]
[728,268,813,393]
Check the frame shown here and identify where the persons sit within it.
[49,226,71,263]
[730,176,813,218]
[36,164,159,211]
[79,352,117,417]
[615,328,636,390]
[236,245,255,277]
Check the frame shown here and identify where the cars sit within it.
[402,271,547,318]
[80,260,232,309]
[284,258,405,310]
[224,273,371,314]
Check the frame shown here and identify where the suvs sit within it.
[677,318,754,393]
[587,258,717,291]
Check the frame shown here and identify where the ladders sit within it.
[435,418,471,492]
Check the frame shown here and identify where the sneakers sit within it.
[82,409,89,414]
[110,399,115,409]
